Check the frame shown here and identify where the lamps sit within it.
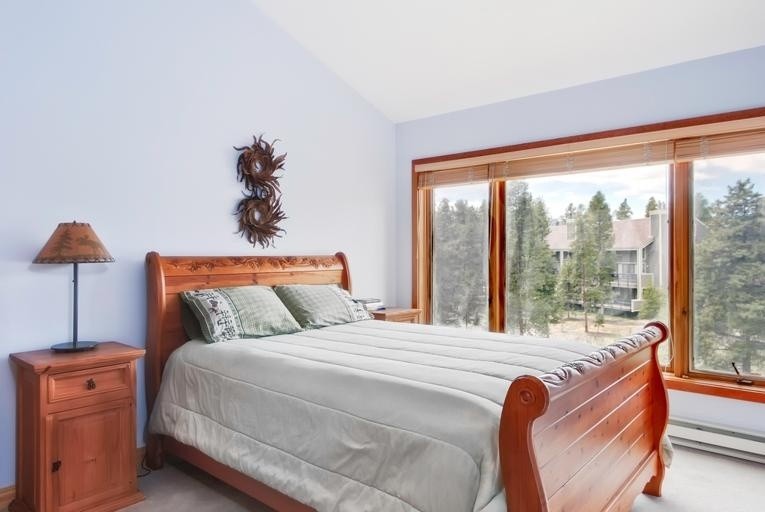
[31,221,116,353]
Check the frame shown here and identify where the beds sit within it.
[144,250,670,510]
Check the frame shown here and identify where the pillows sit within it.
[274,284,374,330]
[178,285,303,343]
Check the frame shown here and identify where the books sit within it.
[355,297,384,311]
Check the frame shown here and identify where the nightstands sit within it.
[9,342,147,512]
[373,308,423,323]
